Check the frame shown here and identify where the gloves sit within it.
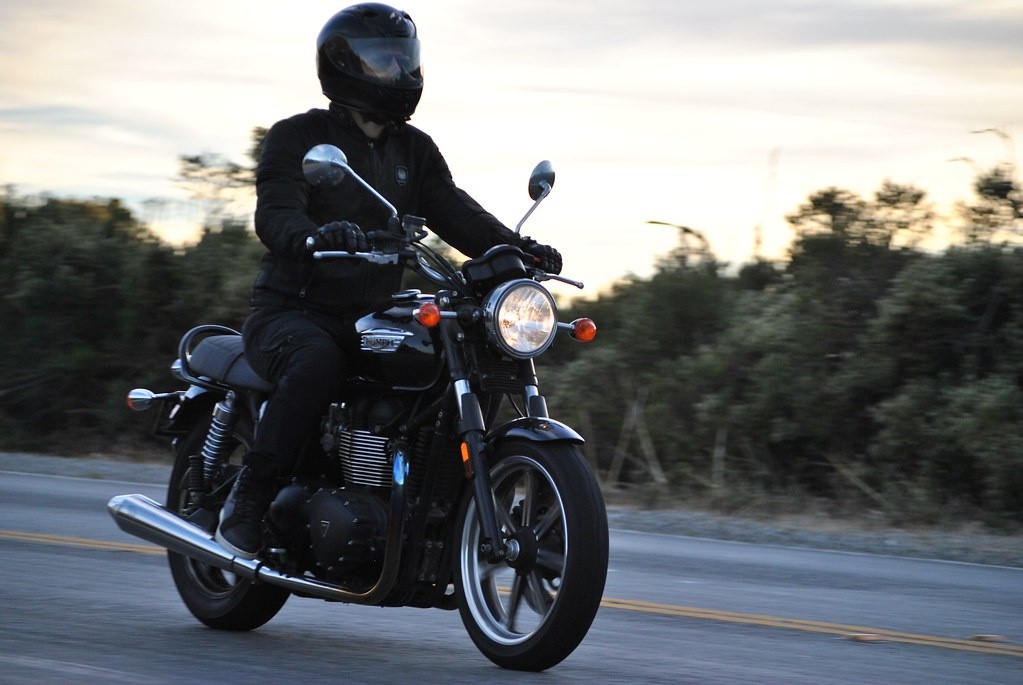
[524,243,563,282]
[313,221,370,254]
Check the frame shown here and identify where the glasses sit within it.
[359,52,412,73]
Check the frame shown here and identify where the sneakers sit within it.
[215,466,276,560]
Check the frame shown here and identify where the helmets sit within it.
[316,3,424,117]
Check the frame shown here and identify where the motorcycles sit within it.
[105,142,609,674]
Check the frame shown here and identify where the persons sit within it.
[215,3,562,561]
